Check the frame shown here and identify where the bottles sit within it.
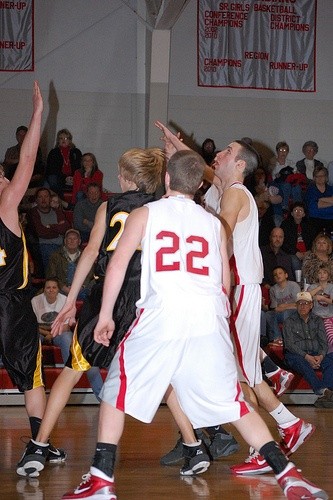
[318,287,330,307]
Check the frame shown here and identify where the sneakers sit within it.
[207,434,240,460]
[274,462,330,500]
[277,417,316,456]
[265,365,295,400]
[269,338,284,347]
[15,439,50,478]
[61,465,118,500]
[46,443,68,464]
[179,439,210,476]
[229,447,274,475]
[160,428,212,465]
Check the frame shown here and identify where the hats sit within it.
[65,229,80,237]
[295,291,312,302]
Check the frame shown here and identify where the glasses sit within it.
[297,301,310,305]
[58,137,71,141]
[279,149,286,152]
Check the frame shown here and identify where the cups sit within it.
[295,270,302,282]
[304,283,310,292]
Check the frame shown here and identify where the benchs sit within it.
[0,188,114,390]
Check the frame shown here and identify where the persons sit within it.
[193,137,333,408]
[31,278,73,365]
[3,126,103,300]
[61,150,329,500]
[154,120,316,475]
[16,132,210,477]
[0,80,68,463]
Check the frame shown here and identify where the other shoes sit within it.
[323,389,333,402]
[313,397,333,409]
